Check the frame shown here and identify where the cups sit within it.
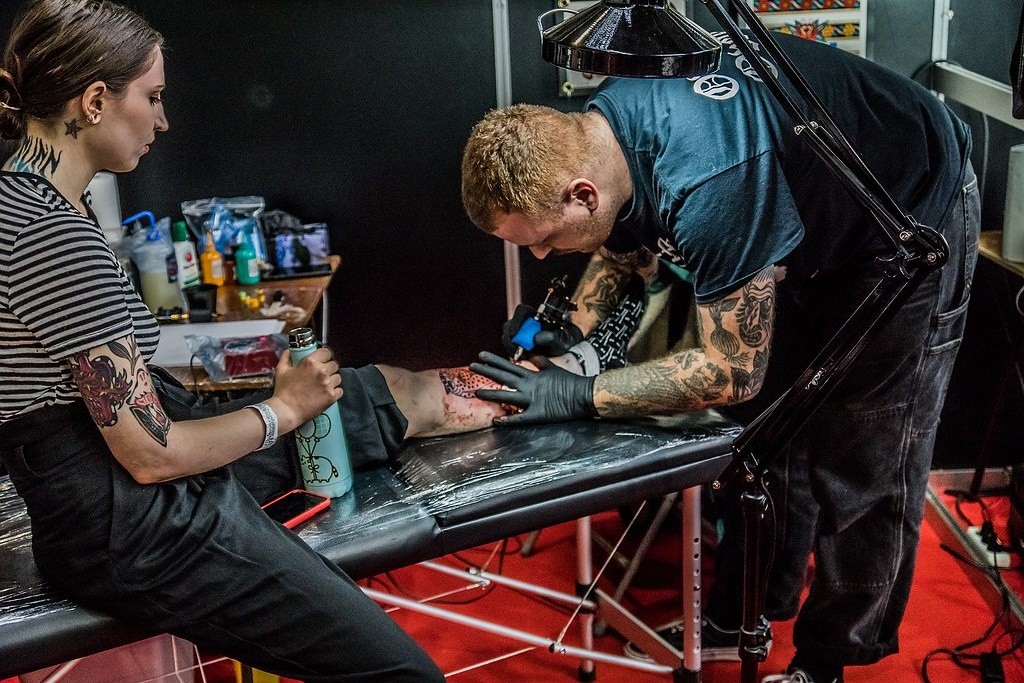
[180,282,218,323]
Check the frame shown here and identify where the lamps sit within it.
[538,0,956,533]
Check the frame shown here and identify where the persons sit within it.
[460,26,982,683]
[1,0,652,682]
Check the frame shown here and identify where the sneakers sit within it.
[761,668,844,683]
[623,616,772,666]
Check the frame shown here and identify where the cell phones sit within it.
[260,487,329,529]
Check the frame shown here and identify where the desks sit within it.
[144,255,343,388]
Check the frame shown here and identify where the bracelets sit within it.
[241,403,280,452]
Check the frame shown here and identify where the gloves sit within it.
[468,348,598,432]
[501,304,584,359]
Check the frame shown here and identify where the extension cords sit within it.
[967,524,1011,568]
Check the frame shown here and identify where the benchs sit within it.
[0,367,745,683]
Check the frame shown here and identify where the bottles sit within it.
[286,327,352,501]
[235,227,259,285]
[134,233,178,315]
[200,227,223,287]
